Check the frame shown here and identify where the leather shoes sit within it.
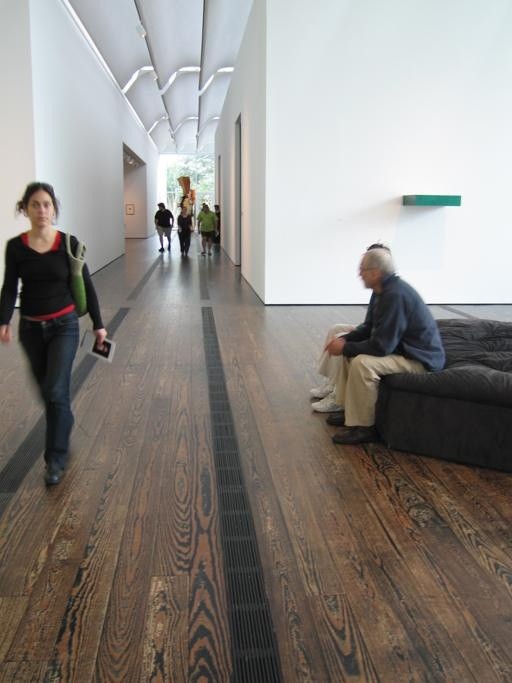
[44,468,64,485]
[332,426,381,445]
[326,413,344,426]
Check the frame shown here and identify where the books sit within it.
[80,329,117,362]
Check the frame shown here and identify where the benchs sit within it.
[374,318,512,474]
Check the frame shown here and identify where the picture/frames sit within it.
[125,204,135,215]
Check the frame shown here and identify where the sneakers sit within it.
[310,382,345,413]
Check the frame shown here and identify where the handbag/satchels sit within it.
[69,252,87,315]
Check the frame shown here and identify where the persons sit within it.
[1,180,117,486]
[154,202,174,251]
[214,203,221,243]
[177,206,194,256]
[201,201,207,210]
[196,205,220,255]
[321,247,446,444]
[310,242,392,415]
